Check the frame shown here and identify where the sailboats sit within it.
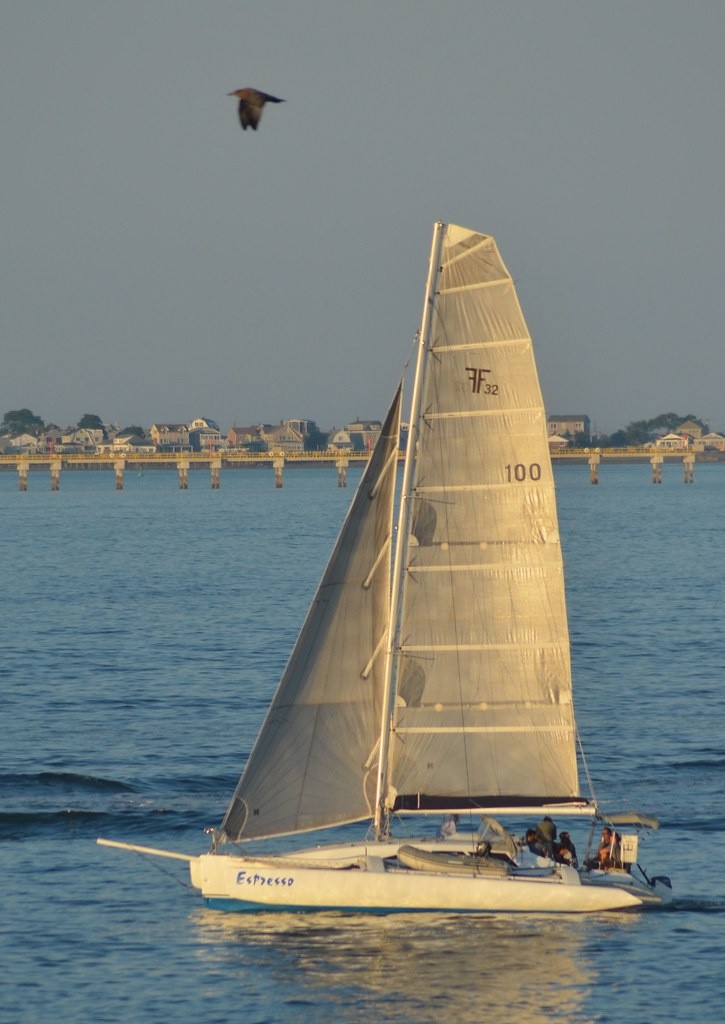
[95,222,671,913]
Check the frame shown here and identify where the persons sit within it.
[524,817,578,867]
[586,827,630,871]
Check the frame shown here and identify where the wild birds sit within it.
[227,88,285,130]
